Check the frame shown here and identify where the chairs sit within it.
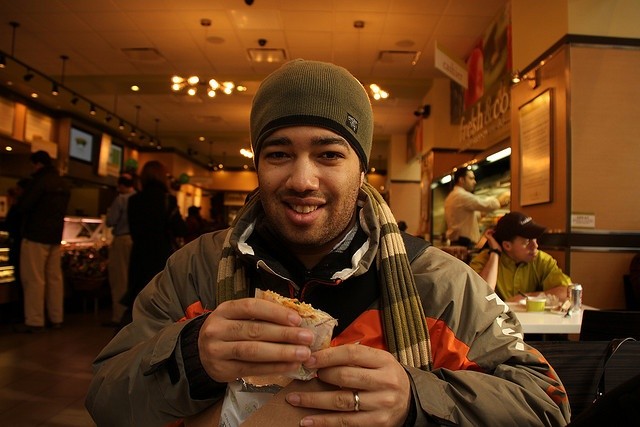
[579,309,640,340]
[523,341,639,427]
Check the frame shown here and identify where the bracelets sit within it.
[488,248,503,256]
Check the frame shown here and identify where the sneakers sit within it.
[47,322,64,329]
[100,320,120,326]
[26,326,47,333]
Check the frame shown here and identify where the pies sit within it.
[218,285,341,425]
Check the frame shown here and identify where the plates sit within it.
[519,299,563,310]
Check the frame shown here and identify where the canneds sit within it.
[566,282,583,309]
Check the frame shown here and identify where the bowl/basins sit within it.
[525,298,546,313]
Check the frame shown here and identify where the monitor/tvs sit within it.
[70,124,95,165]
[107,142,124,178]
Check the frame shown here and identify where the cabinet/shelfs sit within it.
[0,93,59,178]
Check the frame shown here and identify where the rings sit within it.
[353,391,361,413]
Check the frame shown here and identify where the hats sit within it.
[250,57,374,173]
[493,212,549,239]
[187,205,202,214]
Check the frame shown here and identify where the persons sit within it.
[127,161,186,307]
[469,211,574,304]
[18,150,71,335]
[83,58,573,427]
[187,206,209,234]
[444,166,511,250]
[0,177,32,320]
[102,172,138,327]
[209,212,231,233]
